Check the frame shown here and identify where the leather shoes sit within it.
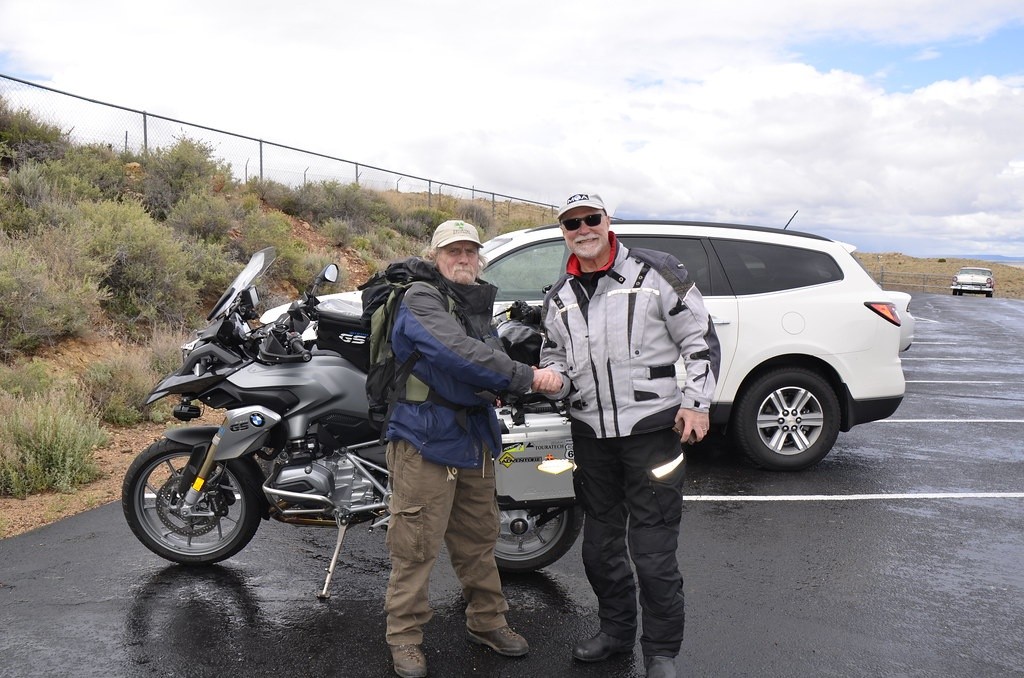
[644,654,676,678]
[572,630,636,662]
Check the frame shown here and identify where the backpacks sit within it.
[358,263,454,445]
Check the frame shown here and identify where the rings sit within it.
[702,428,707,432]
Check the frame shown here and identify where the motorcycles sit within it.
[122,247,585,578]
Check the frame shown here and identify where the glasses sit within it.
[560,213,602,230]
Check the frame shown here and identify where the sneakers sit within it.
[390,644,427,678]
[466,625,529,656]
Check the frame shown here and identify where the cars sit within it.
[950,267,994,298]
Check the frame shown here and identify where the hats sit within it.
[431,220,484,250]
[556,193,608,219]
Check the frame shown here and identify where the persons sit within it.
[382,218,563,678]
[531,191,721,678]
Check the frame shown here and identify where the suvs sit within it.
[259,219,916,469]
